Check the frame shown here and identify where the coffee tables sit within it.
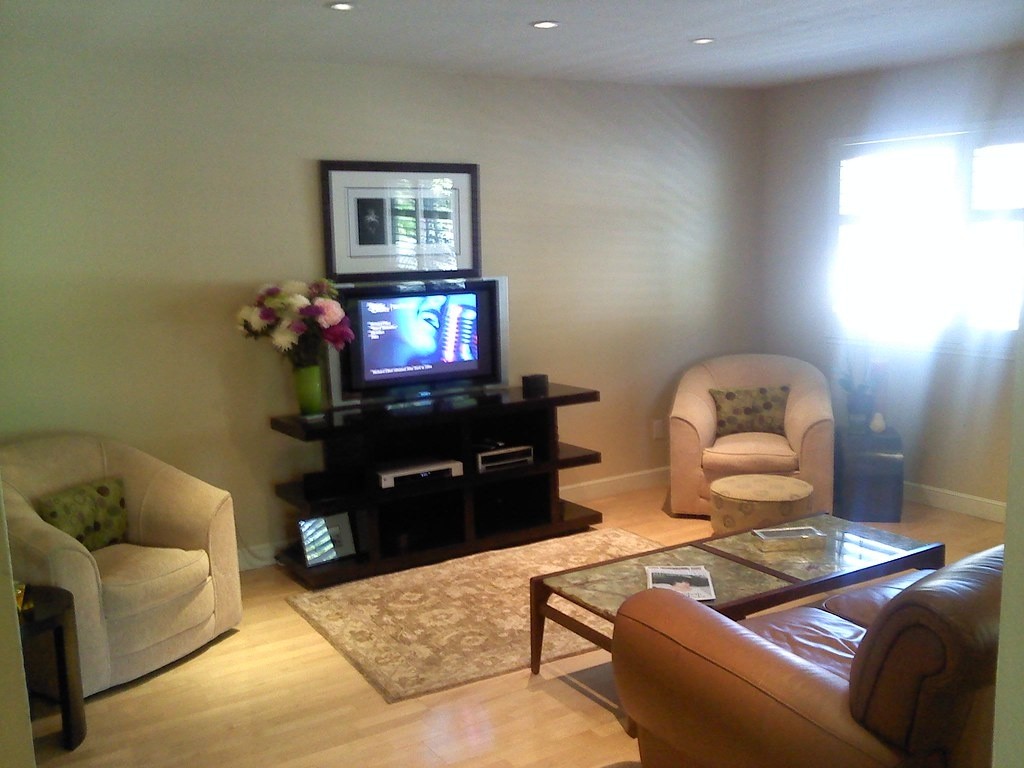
[530,510,945,739]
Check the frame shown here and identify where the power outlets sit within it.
[654,420,663,438]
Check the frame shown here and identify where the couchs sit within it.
[0,433,242,703]
[612,537,1007,768]
[669,353,835,516]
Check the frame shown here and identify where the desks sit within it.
[17,586,86,750]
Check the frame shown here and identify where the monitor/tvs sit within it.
[326,276,509,420]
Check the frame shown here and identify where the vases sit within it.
[295,364,322,417]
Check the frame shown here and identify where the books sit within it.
[751,526,827,552]
[642,564,717,604]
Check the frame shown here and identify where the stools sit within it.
[710,474,815,536]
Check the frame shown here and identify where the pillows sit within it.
[710,382,789,437]
[39,476,128,552]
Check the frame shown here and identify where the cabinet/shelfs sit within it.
[833,423,904,522]
[271,381,603,590]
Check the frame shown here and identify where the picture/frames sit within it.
[298,511,359,566]
[321,159,483,283]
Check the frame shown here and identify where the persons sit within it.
[389,295,445,369]
[667,574,710,600]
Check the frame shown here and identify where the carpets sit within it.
[284,527,741,704]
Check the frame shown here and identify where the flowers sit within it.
[234,276,354,370]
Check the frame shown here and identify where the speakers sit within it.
[522,373,549,399]
